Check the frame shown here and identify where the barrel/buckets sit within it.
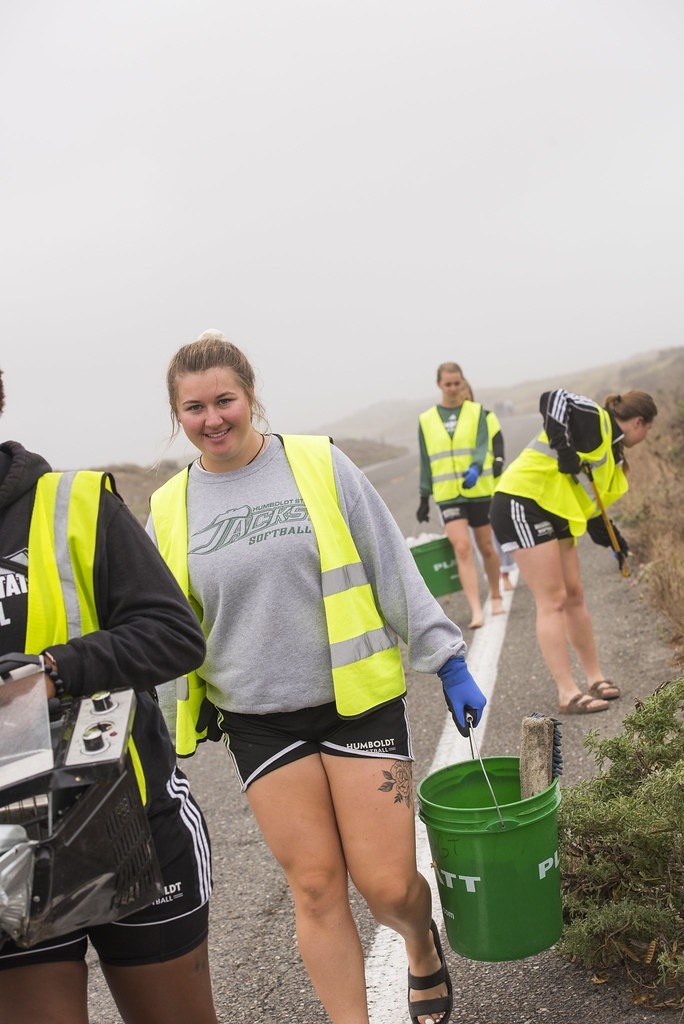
[409,514,463,597]
[415,711,562,961]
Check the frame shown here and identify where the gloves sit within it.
[612,536,628,569]
[437,654,486,738]
[416,495,429,522]
[493,460,502,477]
[558,445,580,475]
[462,464,480,489]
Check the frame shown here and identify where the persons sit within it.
[417,363,516,631]
[489,390,658,713]
[141,329,487,1024]
[0,368,208,1024]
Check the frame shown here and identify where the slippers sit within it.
[589,680,621,700]
[557,693,609,713]
[408,919,452,1024]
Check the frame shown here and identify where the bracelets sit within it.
[44,660,64,698]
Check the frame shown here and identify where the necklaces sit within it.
[200,433,265,471]
